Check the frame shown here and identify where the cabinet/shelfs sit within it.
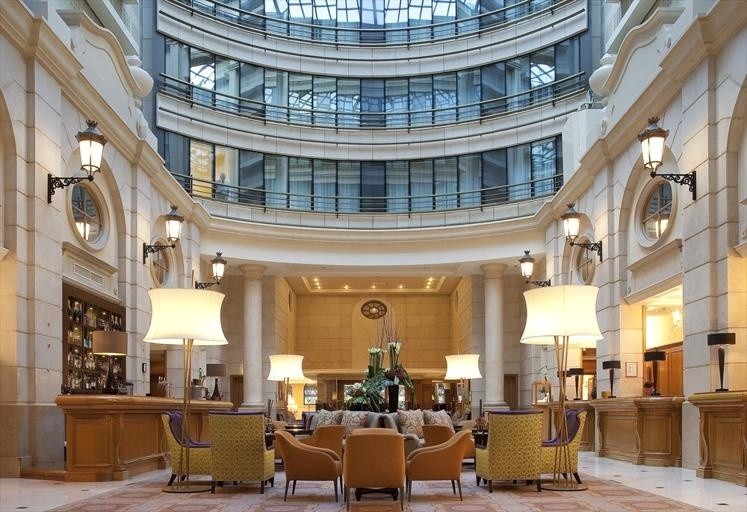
[62,278,126,393]
[545,389,747,488]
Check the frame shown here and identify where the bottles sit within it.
[67,297,123,390]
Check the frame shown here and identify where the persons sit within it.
[215,173,230,200]
[642,380,659,397]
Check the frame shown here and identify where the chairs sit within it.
[160,408,588,512]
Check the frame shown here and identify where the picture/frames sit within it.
[625,362,638,377]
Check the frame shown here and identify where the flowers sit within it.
[341,306,416,412]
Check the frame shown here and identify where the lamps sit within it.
[637,116,697,200]
[142,203,185,264]
[266,354,305,463]
[206,364,227,402]
[443,353,483,421]
[602,360,621,398]
[47,118,108,204]
[569,368,584,400]
[518,284,605,491]
[707,332,736,392]
[142,286,230,493]
[194,250,228,290]
[556,370,571,401]
[643,351,666,397]
[518,249,552,287]
[560,200,603,263]
